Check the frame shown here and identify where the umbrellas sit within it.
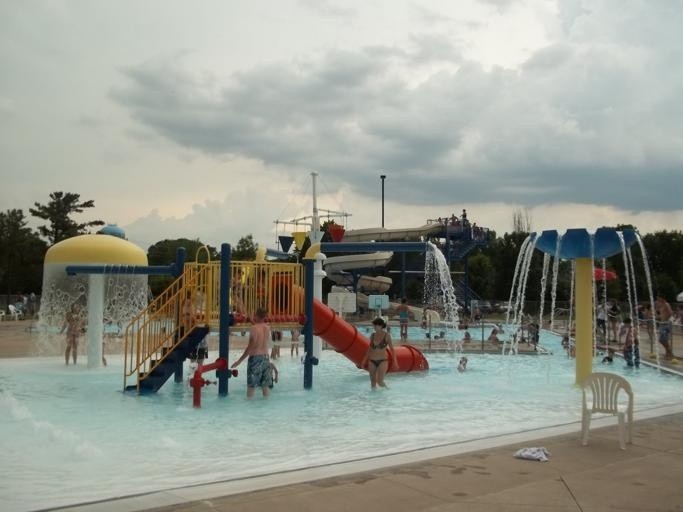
[594,269,616,281]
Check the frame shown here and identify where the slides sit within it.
[298,291,429,373]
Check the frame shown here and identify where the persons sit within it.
[435,207,485,241]
[230,308,271,398]
[58,301,87,366]
[362,316,398,392]
[161,269,300,382]
[387,296,683,372]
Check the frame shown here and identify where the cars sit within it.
[455,299,517,315]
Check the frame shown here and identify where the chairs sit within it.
[582,370,634,451]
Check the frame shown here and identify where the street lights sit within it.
[380,175,386,228]
